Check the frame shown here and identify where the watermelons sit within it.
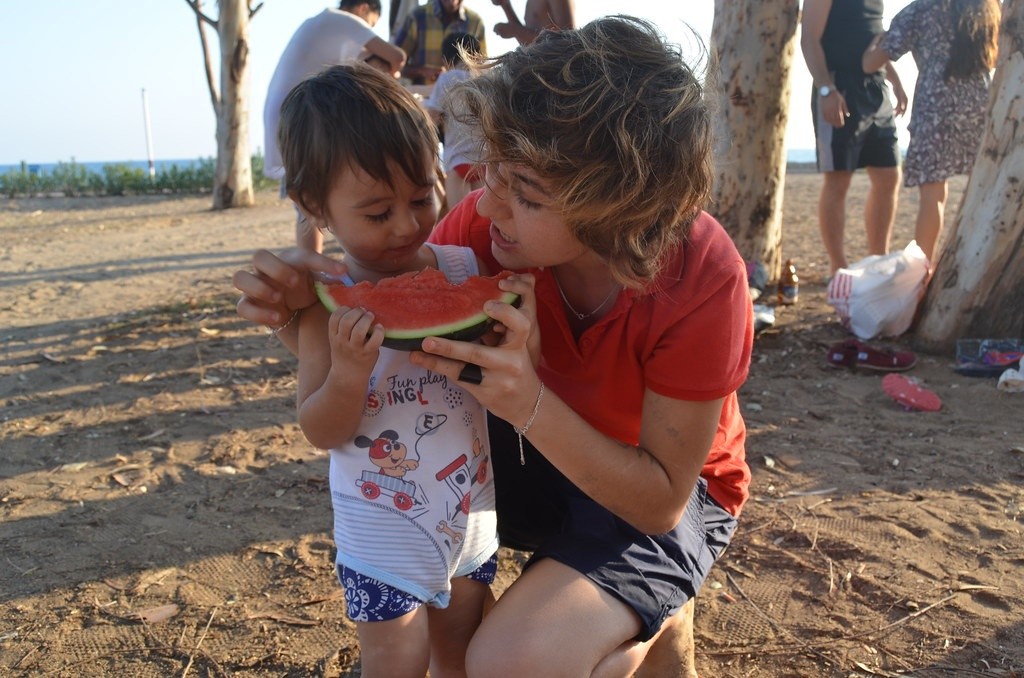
[317,267,521,352]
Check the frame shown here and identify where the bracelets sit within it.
[514,378,545,465]
[265,309,298,333]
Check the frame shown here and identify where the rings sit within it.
[457,363,482,385]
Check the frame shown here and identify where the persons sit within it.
[278,60,552,678]
[490,0,577,49]
[231,13,756,678]
[800,0,909,289]
[862,0,1002,289]
[427,32,487,213]
[263,0,408,255]
[395,0,488,228]
[365,53,391,75]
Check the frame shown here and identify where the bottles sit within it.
[778,259,800,305]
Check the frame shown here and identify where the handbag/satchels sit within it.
[825,240,934,343]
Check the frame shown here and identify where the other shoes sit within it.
[826,339,920,375]
[881,373,942,411]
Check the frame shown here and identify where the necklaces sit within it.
[552,265,618,320]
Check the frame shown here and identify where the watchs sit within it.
[816,83,837,97]
